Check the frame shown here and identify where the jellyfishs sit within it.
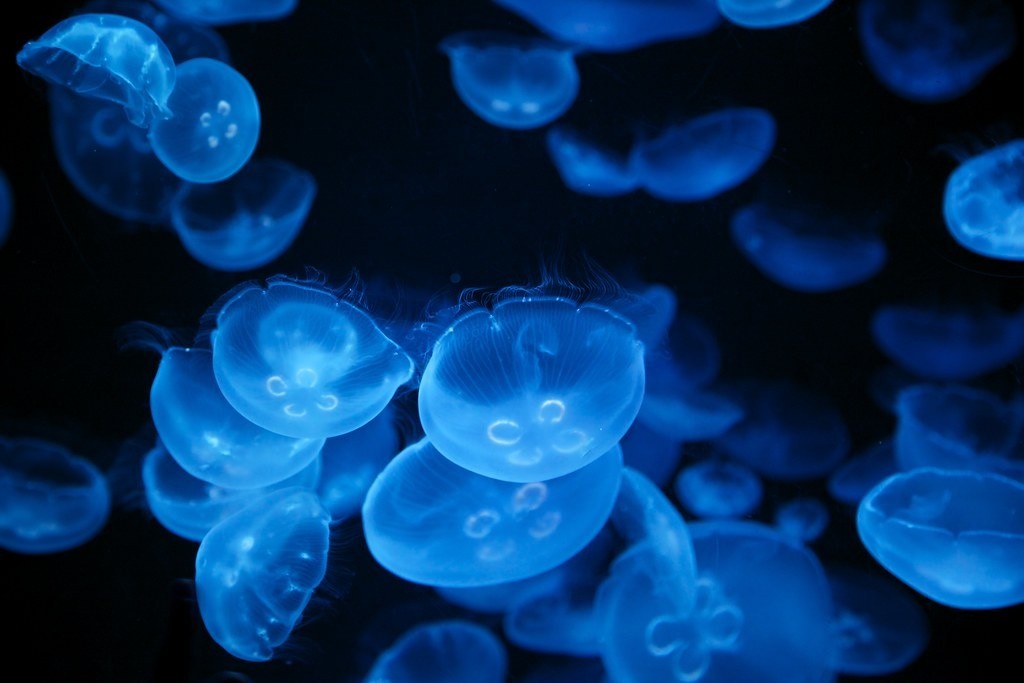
[0,268,1023,682]
[444,0,1024,291]
[18,0,315,271]
